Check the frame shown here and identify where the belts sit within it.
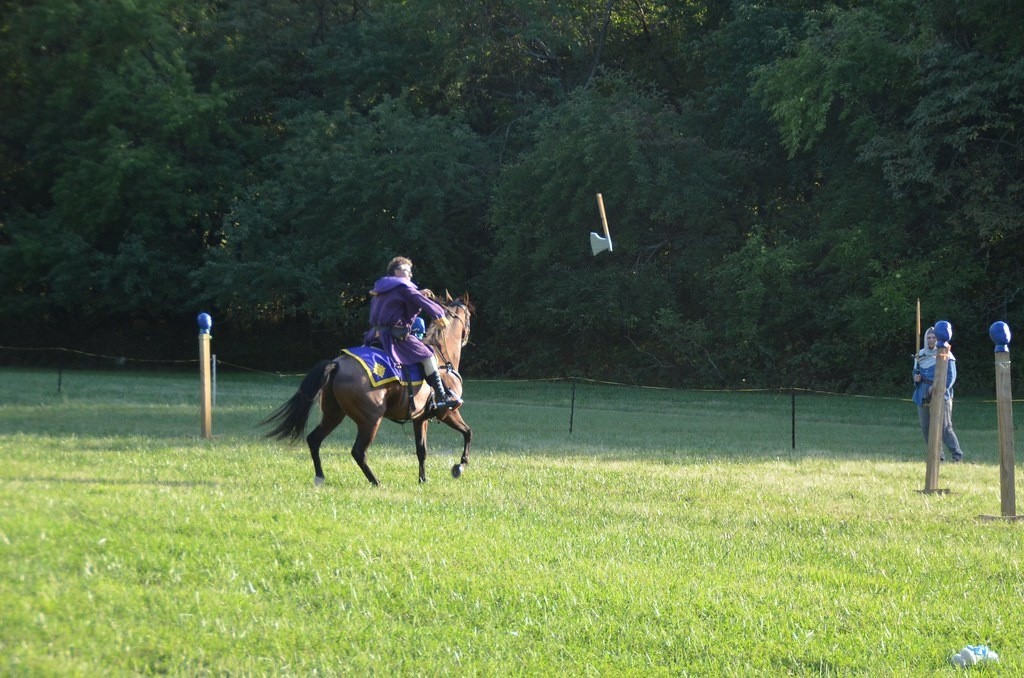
[920,378,934,385]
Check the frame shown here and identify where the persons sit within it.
[365,255,464,421]
[912,326,963,461]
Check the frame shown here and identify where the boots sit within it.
[426,371,462,420]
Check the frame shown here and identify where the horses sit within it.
[254,289,477,489]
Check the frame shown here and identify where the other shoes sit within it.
[952,452,962,461]
